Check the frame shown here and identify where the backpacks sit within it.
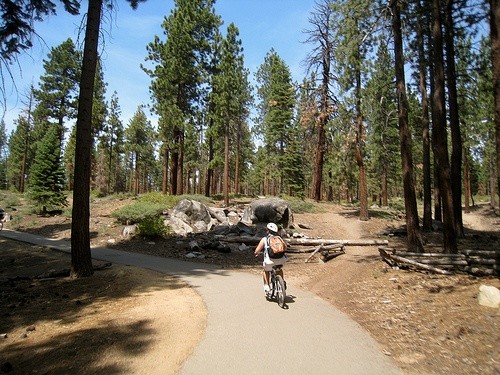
[266,235,288,259]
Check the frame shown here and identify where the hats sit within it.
[263,222,279,233]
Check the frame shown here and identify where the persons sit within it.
[255,223,288,291]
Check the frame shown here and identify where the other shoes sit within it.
[283,280,287,290]
[264,289,272,298]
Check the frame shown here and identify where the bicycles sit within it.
[254,250,288,308]
[0,220,4,232]
